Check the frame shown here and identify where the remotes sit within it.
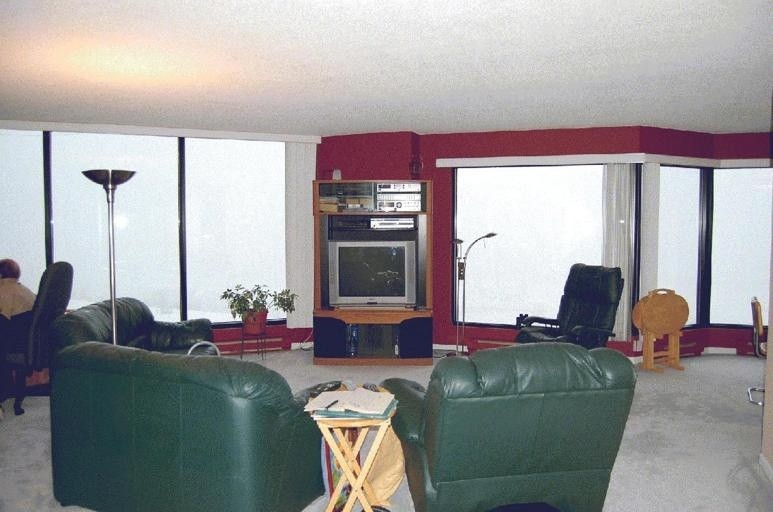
[309,380,341,397]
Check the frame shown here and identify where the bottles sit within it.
[392,335,401,358]
[349,331,360,357]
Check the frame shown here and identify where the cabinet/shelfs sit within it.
[312,179,434,365]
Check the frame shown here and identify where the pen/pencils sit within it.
[325,400,338,408]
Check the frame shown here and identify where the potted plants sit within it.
[218,283,297,334]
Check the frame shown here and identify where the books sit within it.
[301,388,400,422]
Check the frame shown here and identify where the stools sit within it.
[310,384,397,511]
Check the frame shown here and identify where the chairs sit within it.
[749,296,767,358]
[0,258,74,417]
[239,318,266,360]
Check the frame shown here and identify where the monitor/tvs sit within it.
[327,239,417,312]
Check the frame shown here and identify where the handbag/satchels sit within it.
[361,424,406,508]
[321,428,360,512]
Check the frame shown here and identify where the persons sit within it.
[0,257,37,420]
